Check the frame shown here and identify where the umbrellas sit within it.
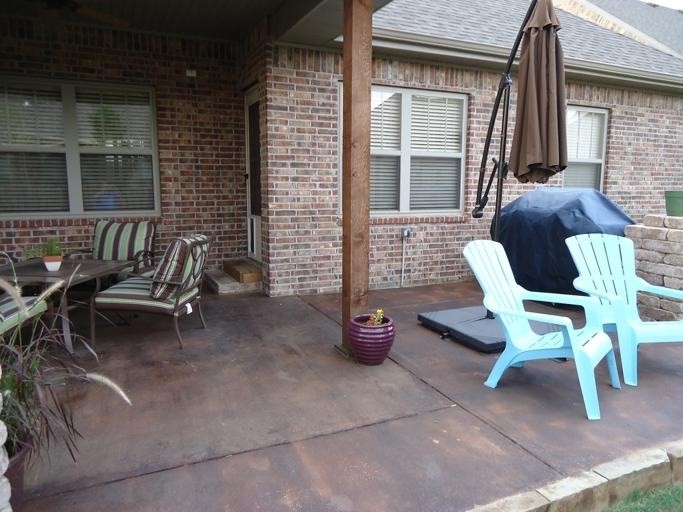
[471,0,569,317]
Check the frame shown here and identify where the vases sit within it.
[348,313,395,366]
[665,190,683,217]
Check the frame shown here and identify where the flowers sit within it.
[0,249,132,512]
[367,304,383,325]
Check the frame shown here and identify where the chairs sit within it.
[463,239,624,418]
[0,221,214,351]
[565,232,683,385]
